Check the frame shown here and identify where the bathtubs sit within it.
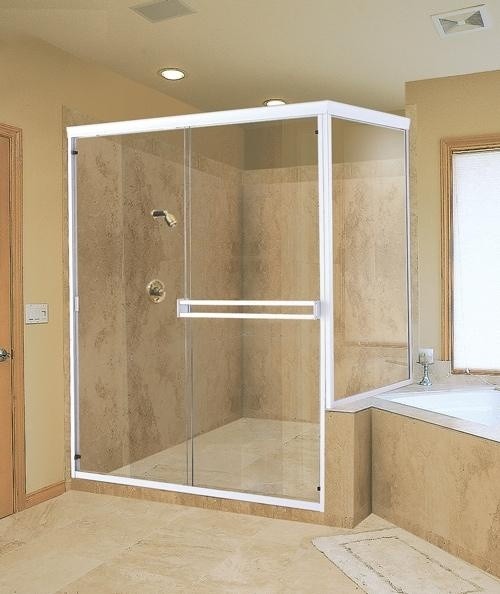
[369,384,500,579]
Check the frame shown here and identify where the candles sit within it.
[419,349,433,364]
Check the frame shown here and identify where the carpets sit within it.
[310,525,499,594]
